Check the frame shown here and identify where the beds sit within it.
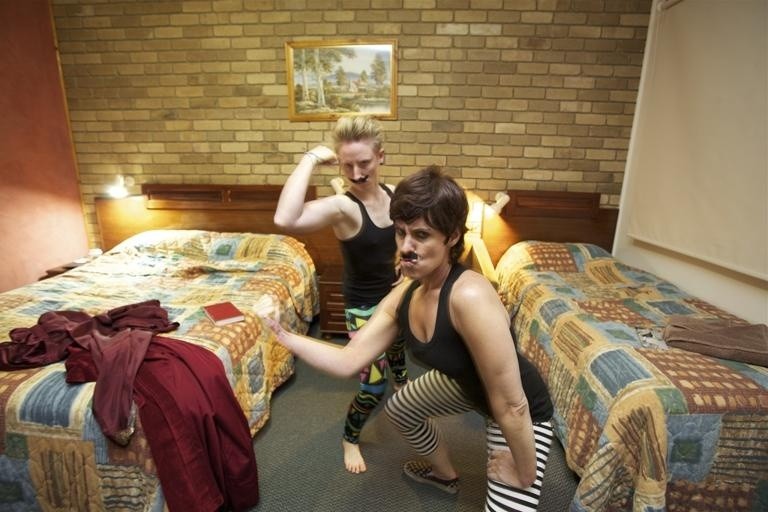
[490,239,766,511]
[0,230,321,511]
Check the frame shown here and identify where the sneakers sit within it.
[404,461,459,494]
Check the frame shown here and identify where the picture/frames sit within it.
[282,36,401,124]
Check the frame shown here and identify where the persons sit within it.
[271,113,415,478]
[253,161,557,511]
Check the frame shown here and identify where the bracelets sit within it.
[304,151,325,166]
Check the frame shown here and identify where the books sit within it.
[200,300,245,329]
